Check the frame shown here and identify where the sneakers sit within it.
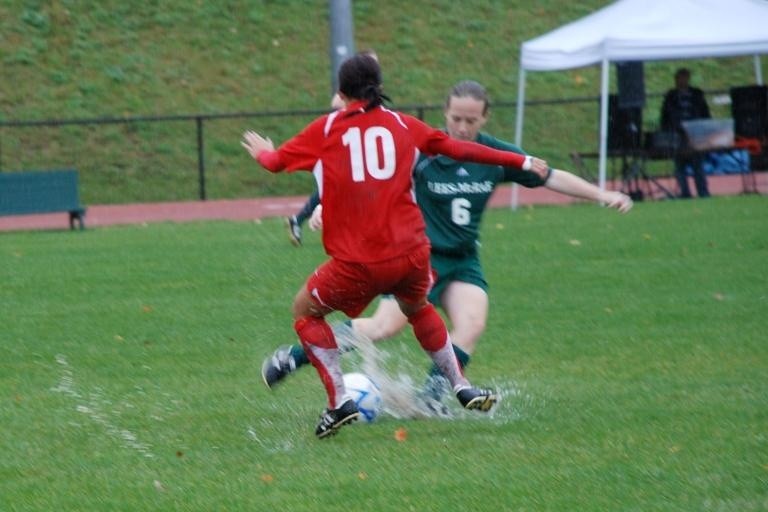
[261,343,295,390]
[284,215,300,248]
[457,386,497,413]
[314,396,360,438]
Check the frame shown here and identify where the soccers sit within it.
[343,373,381,423]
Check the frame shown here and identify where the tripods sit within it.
[613,156,675,202]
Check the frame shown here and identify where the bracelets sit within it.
[522,154,533,172]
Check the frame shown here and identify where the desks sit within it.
[569,138,762,202]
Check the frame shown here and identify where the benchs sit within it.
[0,167,86,231]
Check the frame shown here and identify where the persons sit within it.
[659,67,713,198]
[259,77,636,418]
[239,54,549,441]
[281,47,383,250]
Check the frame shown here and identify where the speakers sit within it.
[729,84,768,141]
[598,94,641,154]
